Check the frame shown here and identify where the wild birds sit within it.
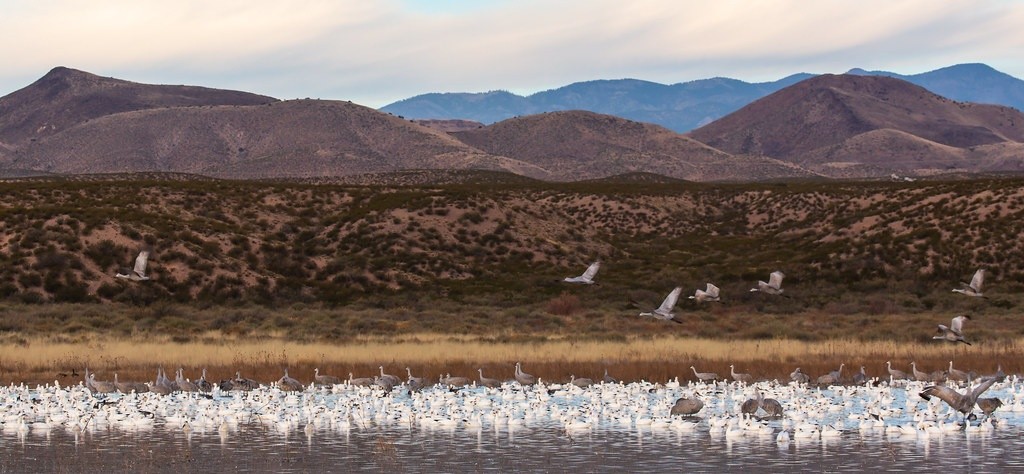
[0,360,1024,447]
[111,250,150,282]
[950,268,990,300]
[560,260,601,287]
[931,314,973,347]
[748,270,785,296]
[687,282,727,306]
[639,286,683,324]
